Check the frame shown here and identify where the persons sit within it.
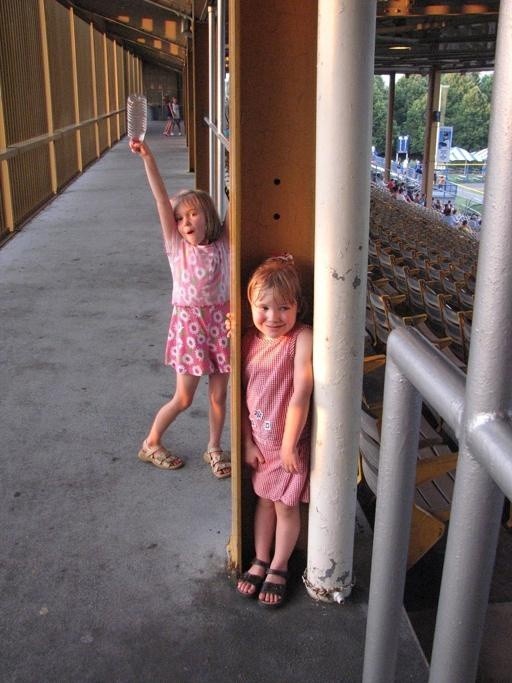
[371,157,481,241]
[443,132,449,147]
[236,254,314,608]
[163,96,175,136]
[129,134,232,478]
[170,96,182,135]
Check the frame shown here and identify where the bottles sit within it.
[125,93,147,151]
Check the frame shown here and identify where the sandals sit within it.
[236,559,270,598]
[138,439,184,470]
[257,568,290,608]
[203,447,231,479]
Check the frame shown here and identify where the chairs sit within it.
[360,183,480,611]
